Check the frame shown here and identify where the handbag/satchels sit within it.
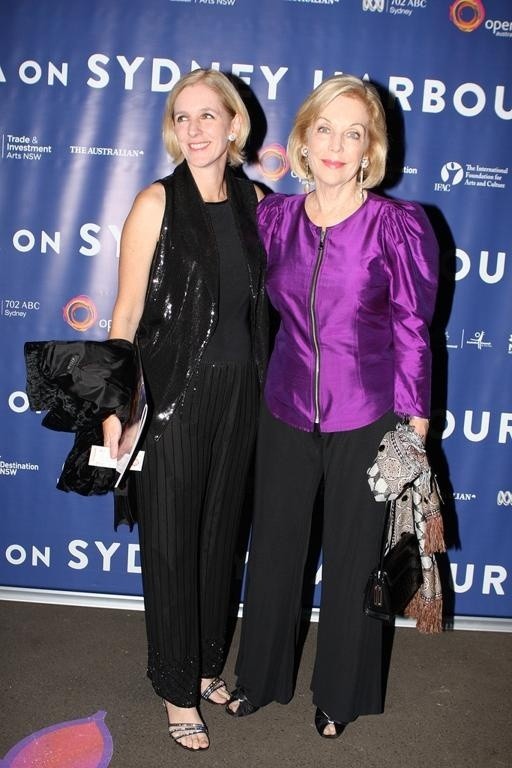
[360,482,426,623]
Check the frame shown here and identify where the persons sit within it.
[101,66,281,752]
[224,73,447,741]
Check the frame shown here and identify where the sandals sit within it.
[199,675,231,707]
[160,695,211,752]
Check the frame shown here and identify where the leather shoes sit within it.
[225,689,262,717]
[313,707,346,739]
